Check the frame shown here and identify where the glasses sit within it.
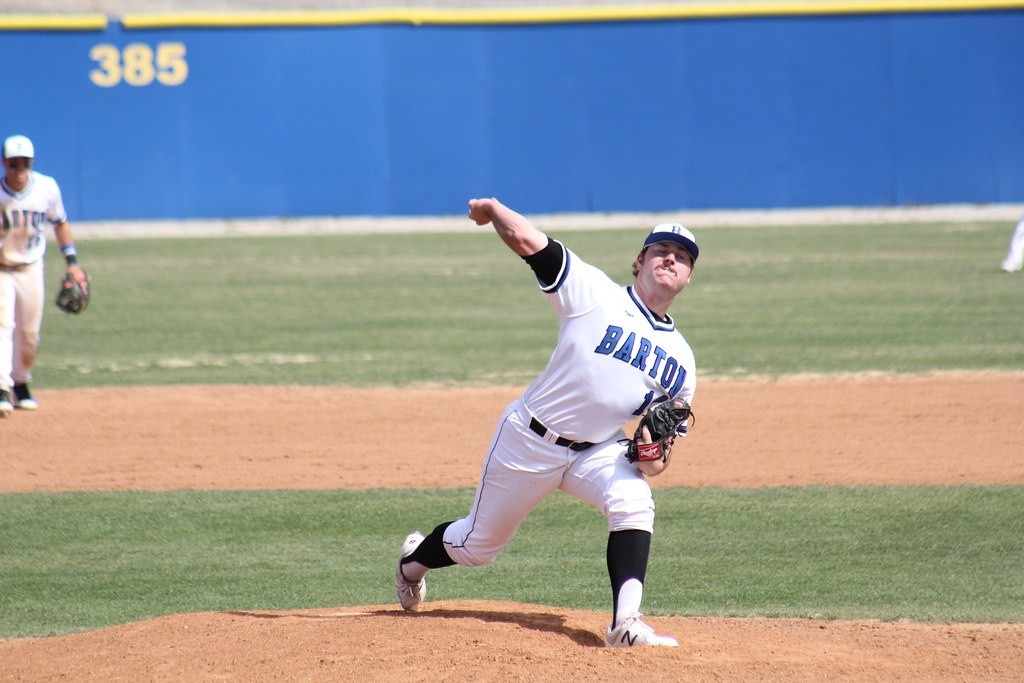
[7,160,32,168]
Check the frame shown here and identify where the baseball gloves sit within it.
[55,265,89,315]
[627,398,691,464]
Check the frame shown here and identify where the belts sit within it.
[529,417,594,451]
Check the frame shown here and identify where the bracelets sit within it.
[61,245,76,257]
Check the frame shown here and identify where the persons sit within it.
[1001,219,1024,272]
[396,196,699,648]
[0,135,90,415]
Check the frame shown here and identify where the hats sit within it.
[643,223,699,266]
[2,135,34,159]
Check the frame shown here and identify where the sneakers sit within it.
[604,613,679,648]
[395,533,426,612]
[0,390,13,411]
[13,383,38,410]
